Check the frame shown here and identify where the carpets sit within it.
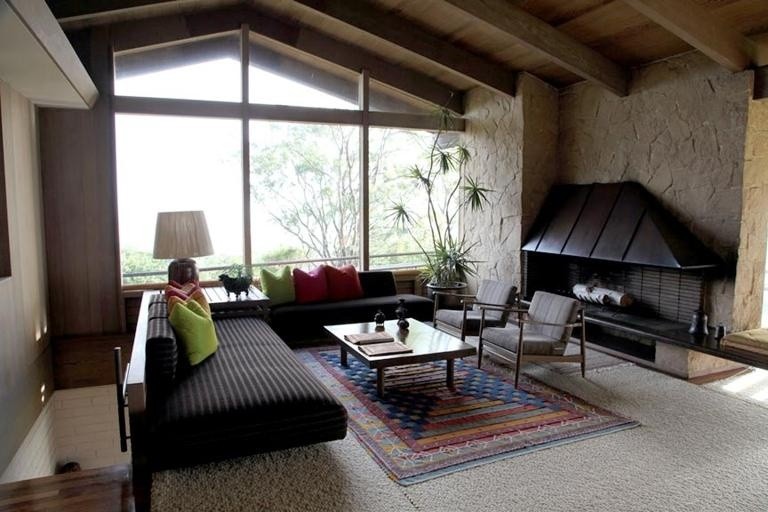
[301,336,644,493]
[144,307,767,511]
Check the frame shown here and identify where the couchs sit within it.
[108,279,352,510]
[255,268,436,351]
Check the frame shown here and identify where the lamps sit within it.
[151,209,215,284]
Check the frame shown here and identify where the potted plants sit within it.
[380,91,498,311]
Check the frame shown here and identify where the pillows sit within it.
[321,261,365,304]
[164,277,220,368]
[258,264,296,308]
[291,263,330,306]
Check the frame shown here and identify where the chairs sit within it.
[475,288,587,390]
[428,278,520,361]
